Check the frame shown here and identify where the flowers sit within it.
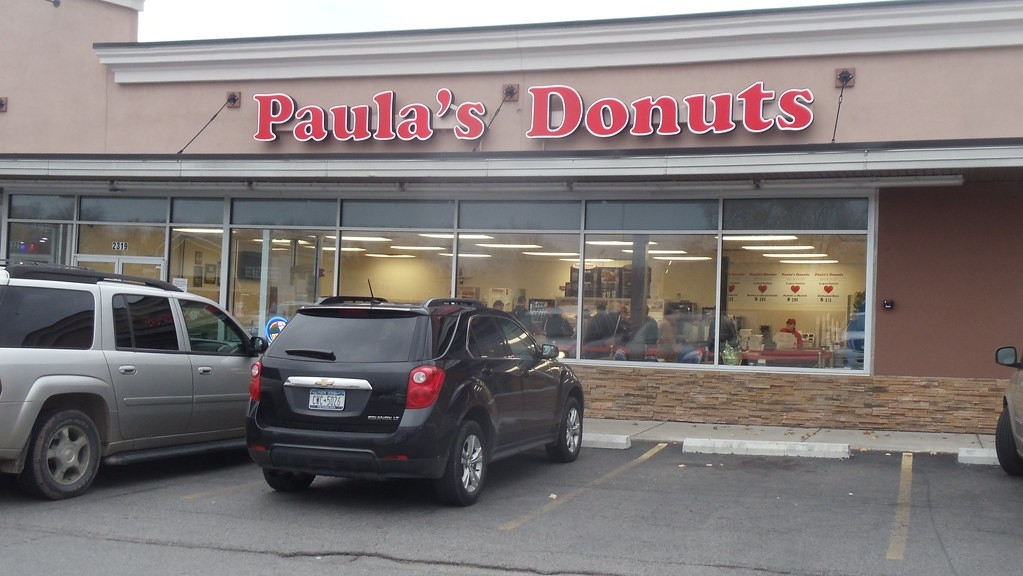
[718,340,744,355]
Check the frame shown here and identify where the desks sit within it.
[707,348,834,368]
[545,342,714,363]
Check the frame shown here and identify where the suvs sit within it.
[0,256,269,501]
[245,278,584,509]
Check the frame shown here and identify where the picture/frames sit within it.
[193,266,203,287]
[195,251,203,265]
[204,263,217,285]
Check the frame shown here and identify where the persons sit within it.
[707,306,738,350]
[659,304,681,342]
[672,335,700,364]
[584,300,631,343]
[541,307,574,338]
[646,305,658,344]
[779,319,802,348]
[492,301,504,311]
[512,296,532,334]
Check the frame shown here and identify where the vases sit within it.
[722,354,742,366]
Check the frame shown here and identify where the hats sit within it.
[786,318,796,324]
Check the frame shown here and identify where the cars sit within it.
[842,312,865,370]
[992,345,1023,484]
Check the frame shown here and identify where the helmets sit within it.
[551,307,561,314]
[608,301,621,314]
